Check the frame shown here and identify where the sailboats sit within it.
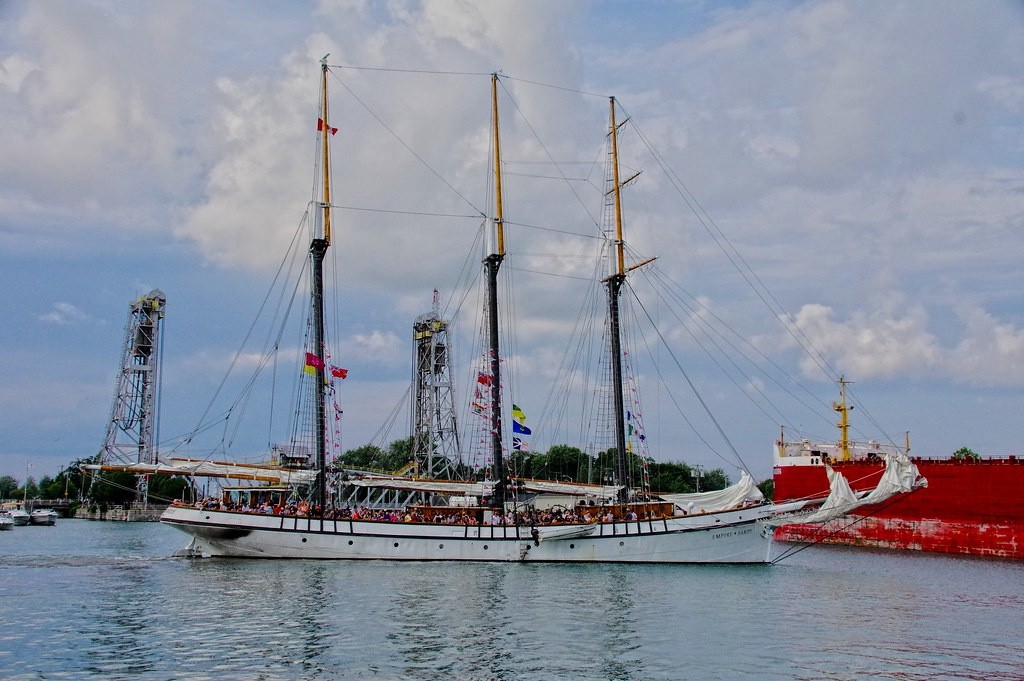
[77,53,929,565]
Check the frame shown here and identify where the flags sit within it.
[319,349,337,396]
[512,404,527,424]
[512,419,532,435]
[304,365,316,377]
[628,423,647,442]
[306,353,326,370]
[624,352,650,500]
[470,348,526,503]
[317,118,339,135]
[477,372,494,387]
[513,437,529,451]
[627,409,642,420]
[321,412,342,466]
[330,365,349,379]
[333,400,343,413]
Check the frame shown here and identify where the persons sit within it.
[198,495,671,526]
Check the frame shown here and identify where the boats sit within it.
[29,508,59,526]
[0,505,15,531]
[7,509,30,527]
[771,374,1024,560]
